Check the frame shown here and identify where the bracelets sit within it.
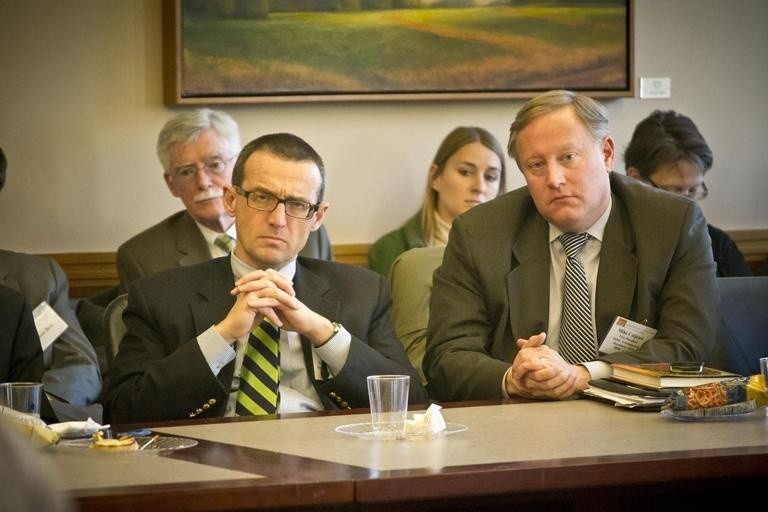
[314,320,340,349]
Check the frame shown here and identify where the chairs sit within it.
[718,275,767,373]
[100,294,128,357]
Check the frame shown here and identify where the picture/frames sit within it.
[164,1,639,106]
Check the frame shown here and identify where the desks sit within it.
[46,397,768,510]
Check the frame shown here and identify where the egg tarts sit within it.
[87,429,138,451]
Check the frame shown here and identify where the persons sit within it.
[1,284,61,424]
[623,111,754,277]
[366,125,507,279]
[0,145,103,426]
[420,88,720,400]
[113,107,333,291]
[100,132,428,424]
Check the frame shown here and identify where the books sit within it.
[576,361,745,414]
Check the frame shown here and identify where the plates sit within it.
[61,434,200,457]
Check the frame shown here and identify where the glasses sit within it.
[168,152,237,182]
[639,173,709,206]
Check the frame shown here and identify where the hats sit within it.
[230,181,321,220]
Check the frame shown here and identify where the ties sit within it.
[234,309,284,418]
[214,232,238,256]
[554,231,599,367]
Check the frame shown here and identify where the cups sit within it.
[0,382,42,427]
[758,358,768,392]
[366,373,411,435]
